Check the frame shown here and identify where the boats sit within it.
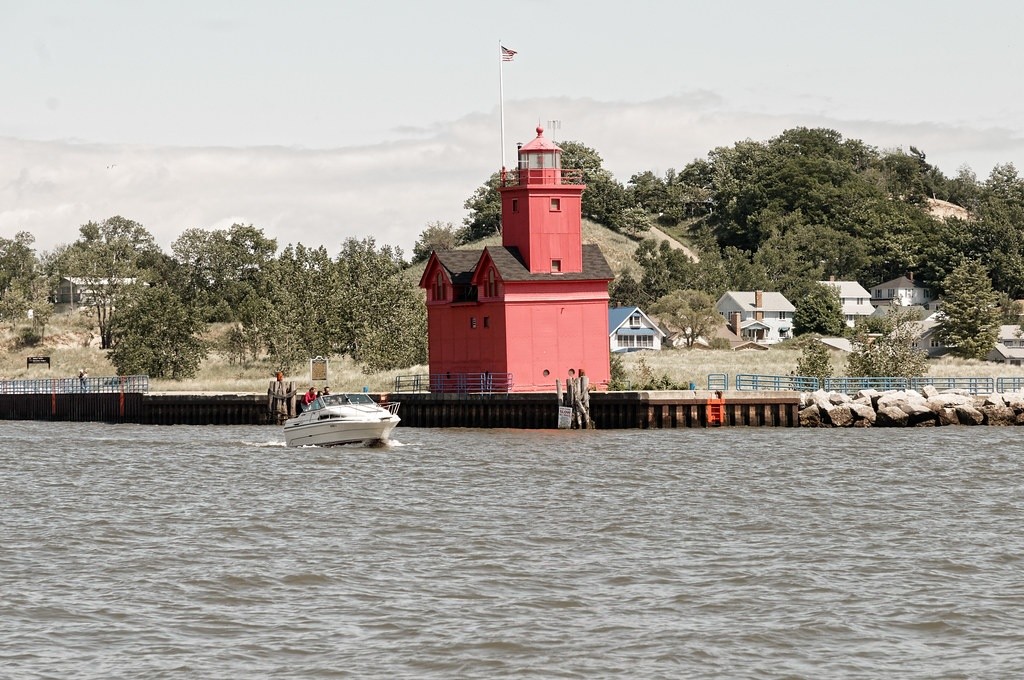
[284,393,402,448]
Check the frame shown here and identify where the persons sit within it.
[79,371,88,392]
[301,387,330,406]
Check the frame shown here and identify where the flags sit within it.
[501,46,517,61]
